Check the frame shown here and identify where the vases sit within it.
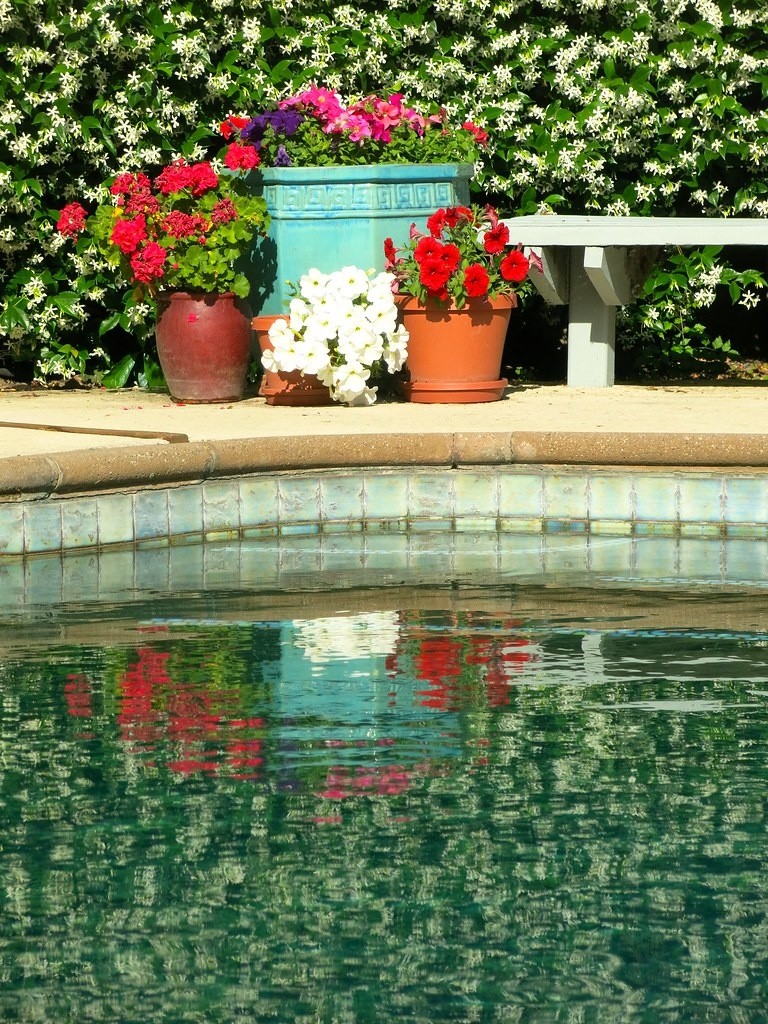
[154,292,257,404]
[253,162,475,316]
[394,295,519,404]
[251,316,337,405]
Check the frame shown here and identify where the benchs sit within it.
[473,214,768,386]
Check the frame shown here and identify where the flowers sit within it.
[384,203,546,311]
[56,157,272,298]
[220,84,491,169]
[260,265,412,407]
